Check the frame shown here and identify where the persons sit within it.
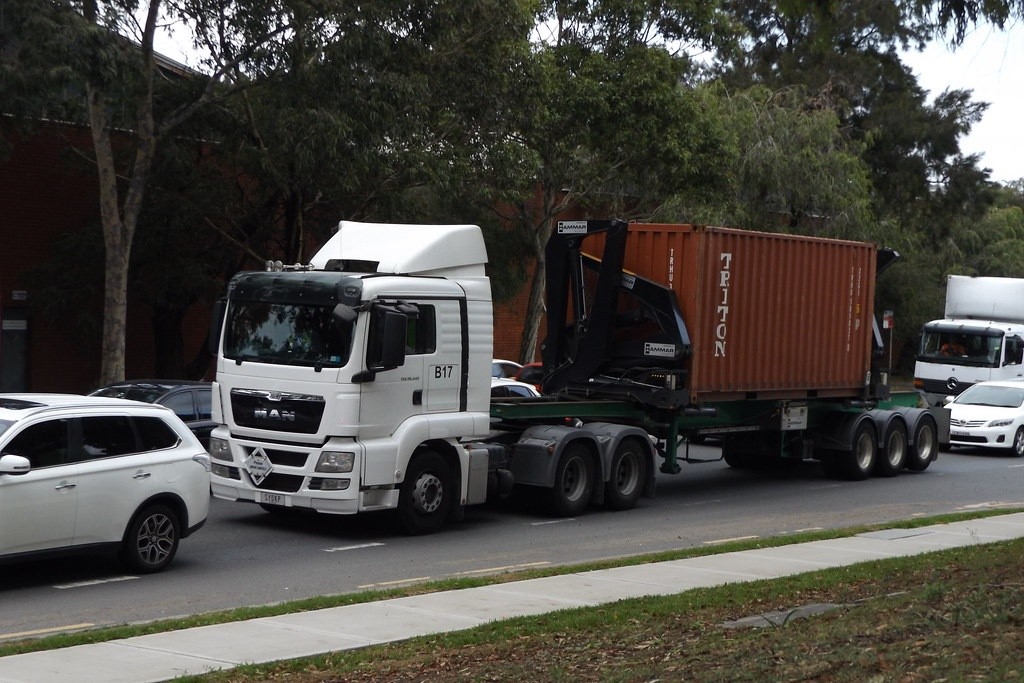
[941,334,966,357]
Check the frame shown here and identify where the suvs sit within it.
[1,392,214,576]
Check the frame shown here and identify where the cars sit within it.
[941,377,1024,457]
[83,379,216,446]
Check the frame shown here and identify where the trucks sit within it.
[916,272,1024,408]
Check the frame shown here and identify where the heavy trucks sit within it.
[209,209,940,532]
